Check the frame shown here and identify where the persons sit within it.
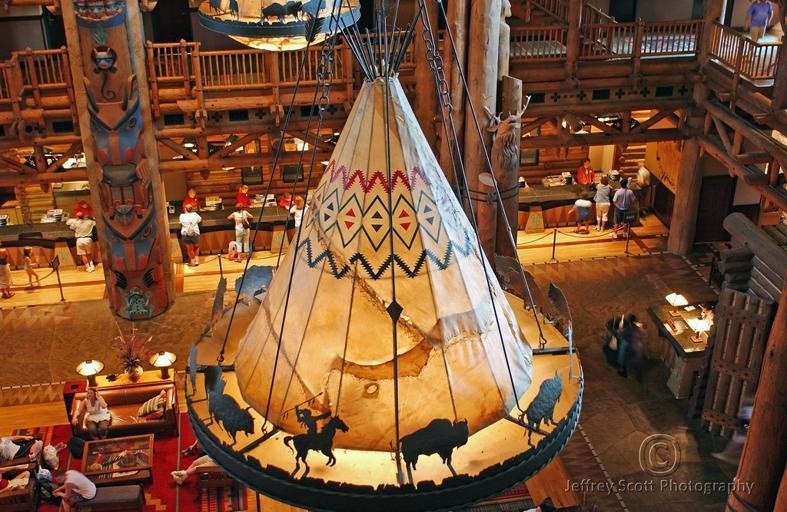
[52,469,96,512]
[70,211,96,273]
[72,388,110,440]
[227,203,253,254]
[290,195,309,234]
[744,0,774,57]
[572,121,589,134]
[170,454,220,485]
[73,200,95,219]
[615,314,649,384]
[568,158,650,233]
[23,246,40,288]
[295,405,331,453]
[235,185,252,208]
[0,436,42,461]
[179,204,202,266]
[279,192,297,210]
[181,439,203,456]
[181,188,206,213]
[0,242,15,298]
[0,464,30,493]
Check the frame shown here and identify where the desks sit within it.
[83,368,174,392]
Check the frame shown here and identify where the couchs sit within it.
[69,384,180,441]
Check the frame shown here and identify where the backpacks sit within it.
[185,221,196,237]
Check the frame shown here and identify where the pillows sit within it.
[138,389,167,420]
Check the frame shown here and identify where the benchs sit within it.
[76,484,146,512]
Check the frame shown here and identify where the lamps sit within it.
[76,360,104,386]
[149,351,176,379]
[686,317,710,343]
[196,0,362,52]
[665,292,688,317]
[185,0,585,512]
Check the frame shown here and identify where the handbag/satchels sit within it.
[243,220,250,229]
[39,478,61,506]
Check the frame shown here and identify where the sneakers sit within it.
[171,470,183,485]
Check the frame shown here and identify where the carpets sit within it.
[11,411,248,512]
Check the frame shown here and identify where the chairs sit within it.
[0,434,46,512]
[196,443,240,495]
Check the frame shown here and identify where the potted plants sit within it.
[106,319,152,383]
